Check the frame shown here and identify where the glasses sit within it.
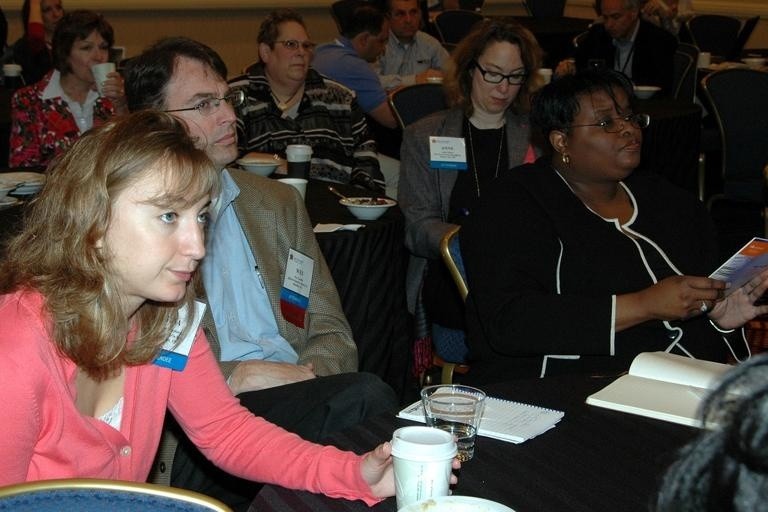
[474,62,525,85]
[275,39,316,51]
[162,89,245,117]
[568,113,650,133]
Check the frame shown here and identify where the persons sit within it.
[652,354,767,512]
[312,1,766,384]
[0,1,461,510]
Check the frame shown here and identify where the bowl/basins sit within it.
[0,183,16,200]
[339,196,396,221]
[632,86,663,99]
[740,57,766,66]
[235,159,281,177]
[399,496,518,512]
[0,173,47,194]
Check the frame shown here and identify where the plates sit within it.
[0,197,18,206]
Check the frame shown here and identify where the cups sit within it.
[535,68,553,87]
[696,51,712,69]
[390,426,458,512]
[286,145,313,181]
[421,384,486,462]
[3,65,22,76]
[278,179,307,203]
[426,77,444,83]
[90,63,116,99]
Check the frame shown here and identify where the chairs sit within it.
[1,477,230,512]
